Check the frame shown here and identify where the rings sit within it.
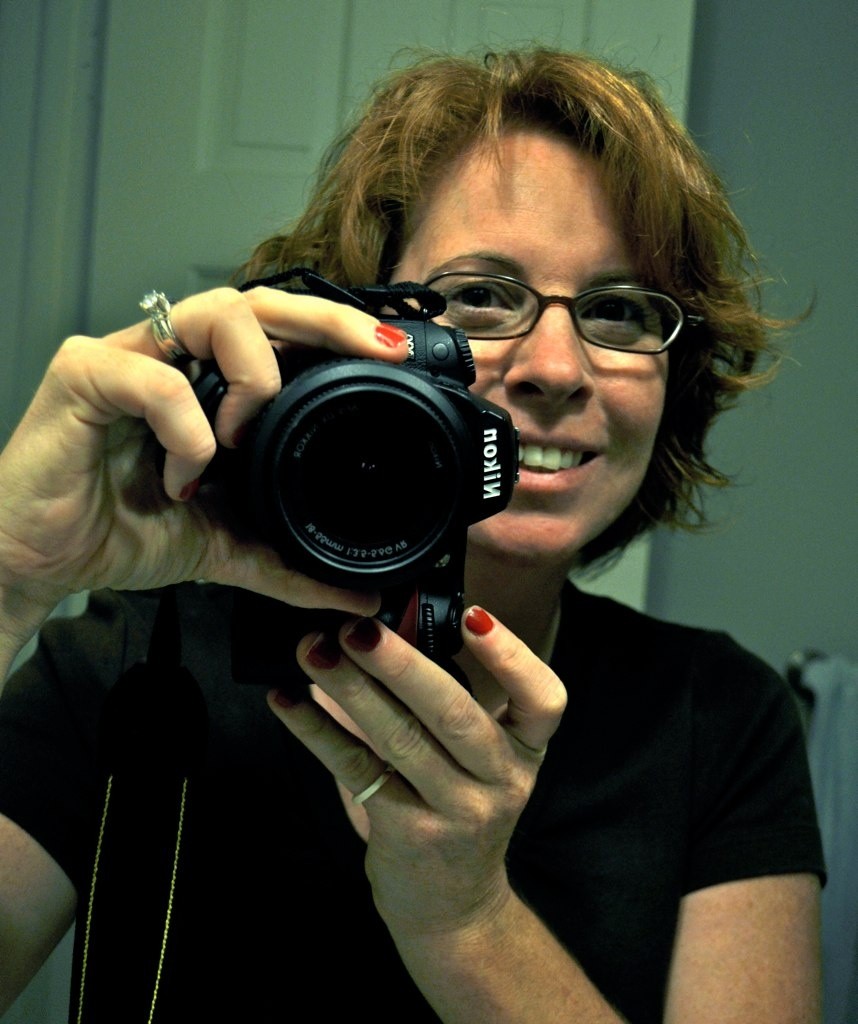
[351,765,395,804]
[138,288,192,364]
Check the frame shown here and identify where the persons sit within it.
[0,40,829,1024]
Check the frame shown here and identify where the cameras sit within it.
[156,317,521,655]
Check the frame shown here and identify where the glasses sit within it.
[422,270,686,354]
